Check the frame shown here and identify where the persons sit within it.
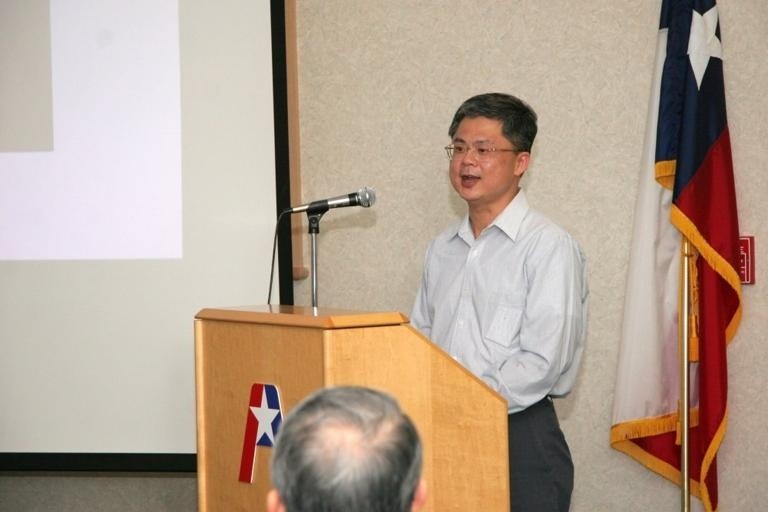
[266,384,427,511]
[409,92,589,511]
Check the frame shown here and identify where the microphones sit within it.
[282,185,377,216]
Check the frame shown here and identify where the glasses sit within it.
[445,144,519,161]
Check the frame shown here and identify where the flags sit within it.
[610,1,744,510]
